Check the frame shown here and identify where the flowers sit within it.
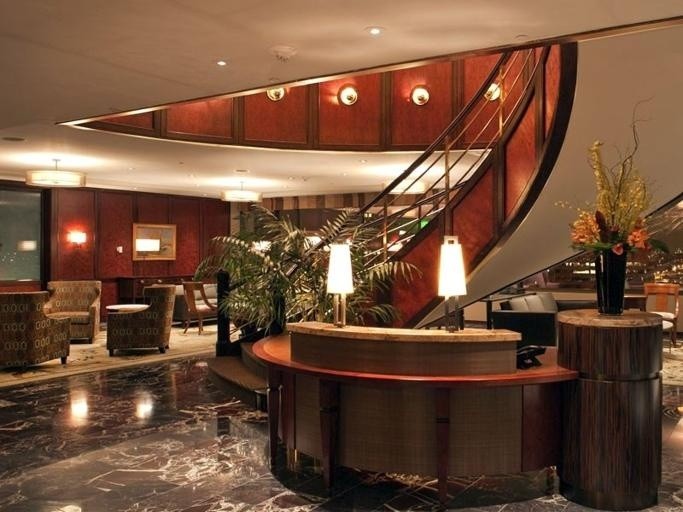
[555,94,671,258]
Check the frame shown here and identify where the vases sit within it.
[596,250,627,316]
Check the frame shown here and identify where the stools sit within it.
[660,320,674,354]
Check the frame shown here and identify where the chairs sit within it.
[183,280,218,335]
[0,279,176,370]
[642,282,681,351]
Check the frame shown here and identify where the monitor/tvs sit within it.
[491,311,557,346]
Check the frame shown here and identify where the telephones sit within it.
[517,345,547,370]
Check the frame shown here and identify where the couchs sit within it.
[173,283,220,322]
[492,288,597,346]
